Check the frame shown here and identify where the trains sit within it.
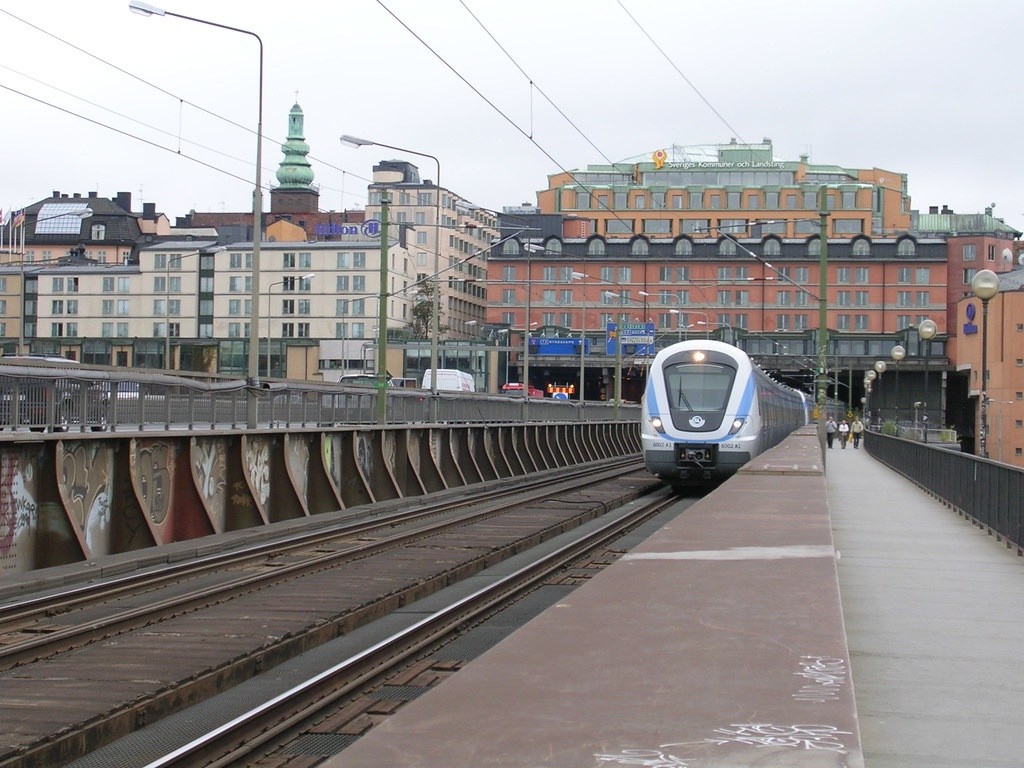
[639,339,845,492]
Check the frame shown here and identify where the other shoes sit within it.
[854,445,859,449]
[828,446,833,449]
[842,447,845,449]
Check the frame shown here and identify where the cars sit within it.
[337,372,411,425]
[0,354,109,433]
[109,375,146,398]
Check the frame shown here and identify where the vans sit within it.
[422,369,476,392]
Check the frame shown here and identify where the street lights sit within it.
[268,273,316,379]
[342,292,380,375]
[604,290,651,381]
[164,246,227,371]
[677,323,695,341]
[875,360,886,433]
[860,369,877,432]
[339,133,441,394]
[571,272,621,421]
[18,206,94,355]
[918,319,937,443]
[891,345,906,437]
[971,268,1000,458]
[523,242,585,421]
[637,291,682,342]
[697,321,733,348]
[129,1,265,427]
[670,310,709,340]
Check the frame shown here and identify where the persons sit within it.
[825,416,837,449]
[851,415,863,449]
[838,418,850,449]
